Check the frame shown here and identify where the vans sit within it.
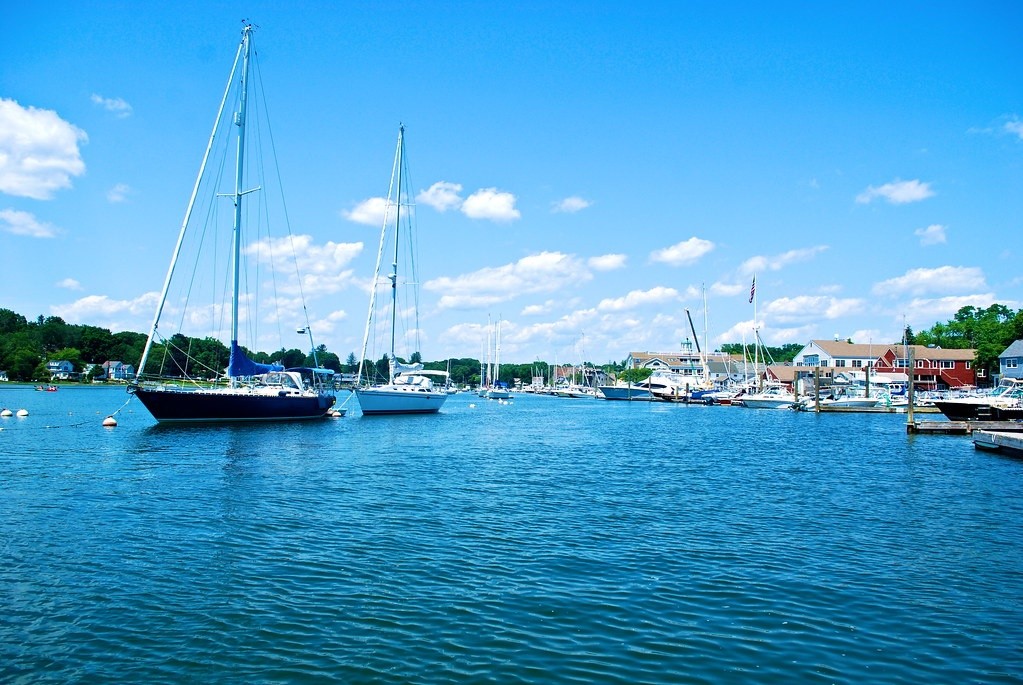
[50,371,79,382]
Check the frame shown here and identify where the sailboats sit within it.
[125,16,337,418]
[445,381,471,394]
[347,121,451,414]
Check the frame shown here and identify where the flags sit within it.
[749,277,756,303]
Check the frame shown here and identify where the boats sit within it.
[33,383,60,392]
[474,271,1023,436]
